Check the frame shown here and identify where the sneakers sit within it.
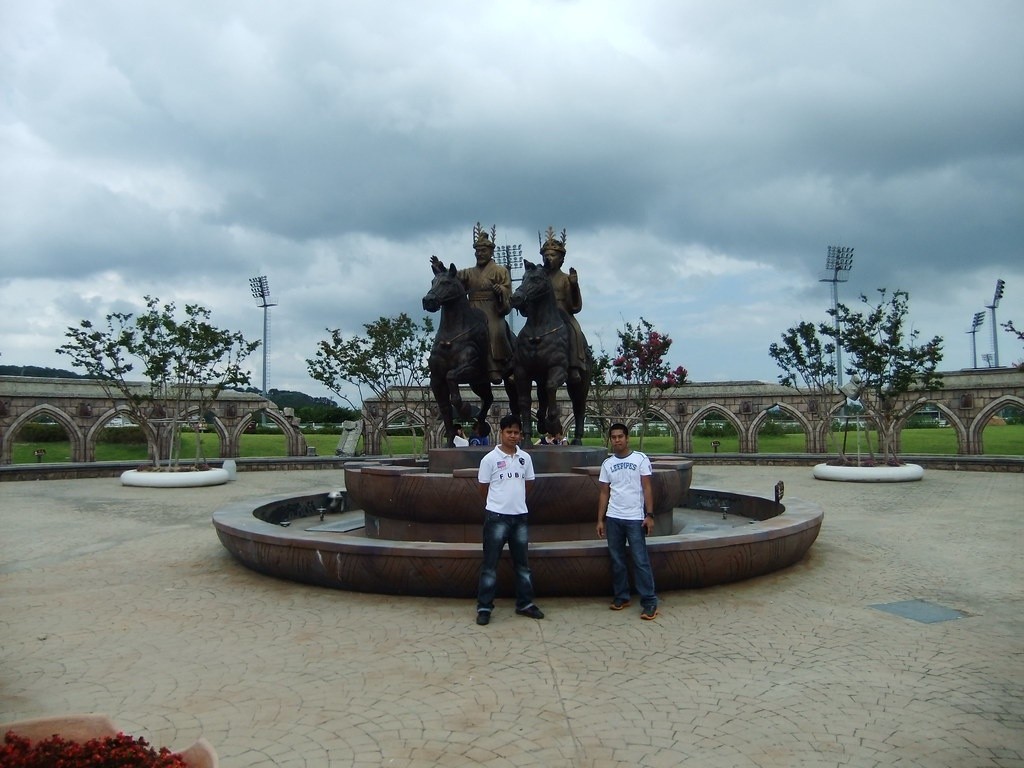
[641,606,658,619]
[609,598,630,611]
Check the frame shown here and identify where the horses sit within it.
[422,259,595,449]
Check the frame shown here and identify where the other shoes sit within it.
[476,611,490,625]
[516,605,543,619]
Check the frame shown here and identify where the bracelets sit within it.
[645,512,655,519]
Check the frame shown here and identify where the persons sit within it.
[533,424,570,445]
[508,237,588,383]
[452,422,491,447]
[595,422,659,620]
[428,236,513,384]
[475,414,545,626]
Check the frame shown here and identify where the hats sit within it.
[453,424,462,429]
